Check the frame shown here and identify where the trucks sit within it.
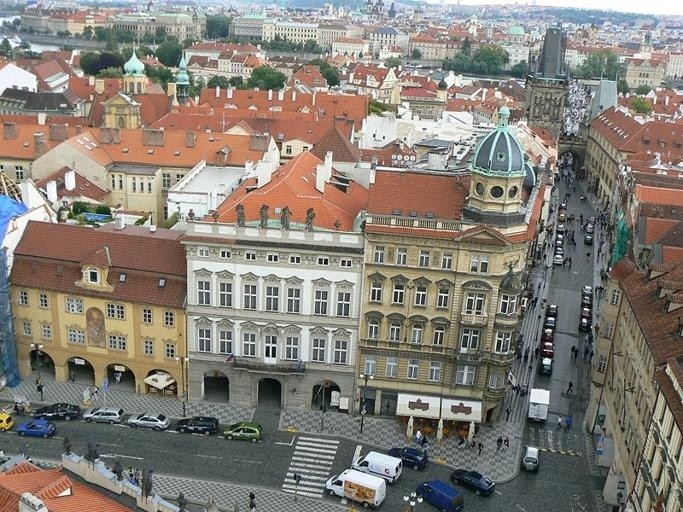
[527,387,550,423]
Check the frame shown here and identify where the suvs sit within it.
[81,406,124,425]
[221,421,262,444]
[175,416,218,437]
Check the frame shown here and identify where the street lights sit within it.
[172,354,193,417]
[356,369,373,433]
[402,490,423,512]
[29,343,46,402]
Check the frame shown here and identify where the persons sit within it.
[89,385,98,400]
[565,415,573,431]
[517,344,540,371]
[502,436,509,451]
[14,396,32,416]
[248,491,256,512]
[558,414,562,424]
[478,443,483,455]
[472,436,476,448]
[570,323,600,363]
[458,436,465,449]
[567,381,573,393]
[506,406,512,421]
[496,436,502,451]
[515,384,520,394]
[127,466,140,485]
[539,158,613,298]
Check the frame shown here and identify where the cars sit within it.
[578,284,592,333]
[127,412,170,432]
[0,412,14,433]
[388,445,431,472]
[34,403,79,421]
[17,419,55,438]
[583,214,596,246]
[536,303,559,375]
[520,443,541,473]
[552,201,566,266]
[450,467,496,499]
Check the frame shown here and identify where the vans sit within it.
[323,468,386,510]
[415,479,464,512]
[350,452,403,485]
